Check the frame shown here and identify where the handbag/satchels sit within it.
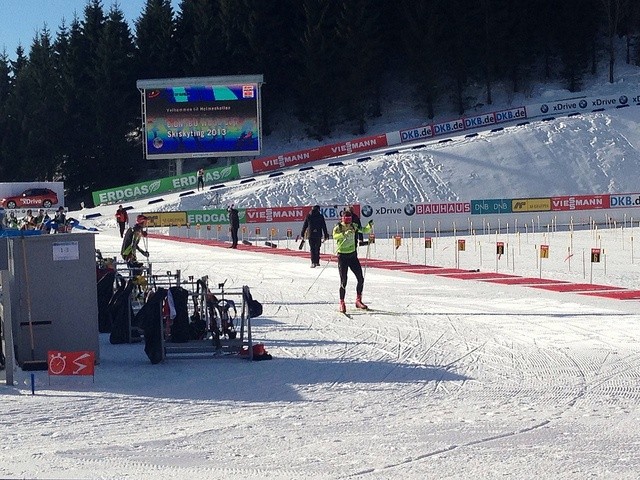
[248,300,262,319]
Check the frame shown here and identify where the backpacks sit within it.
[308,214,322,233]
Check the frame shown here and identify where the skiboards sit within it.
[342,308,375,319]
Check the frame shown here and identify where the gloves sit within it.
[142,251,148,256]
[301,236,304,240]
[366,223,374,228]
[343,230,352,237]
[142,231,147,237]
[324,234,329,240]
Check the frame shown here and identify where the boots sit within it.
[340,299,346,311]
[356,295,367,309]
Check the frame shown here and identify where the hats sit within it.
[341,212,352,223]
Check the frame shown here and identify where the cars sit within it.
[0,188,58,208]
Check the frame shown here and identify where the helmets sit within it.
[136,215,148,224]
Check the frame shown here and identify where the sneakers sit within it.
[253,344,272,361]
[240,348,250,359]
[311,263,316,268]
[230,244,236,249]
[315,263,320,266]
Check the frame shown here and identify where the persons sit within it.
[301,206,330,268]
[54,206,66,225]
[36,209,45,225]
[115,205,129,239]
[121,215,151,269]
[333,211,374,314]
[20,210,36,231]
[196,168,204,190]
[226,205,239,248]
[8,211,18,228]
[349,206,364,247]
